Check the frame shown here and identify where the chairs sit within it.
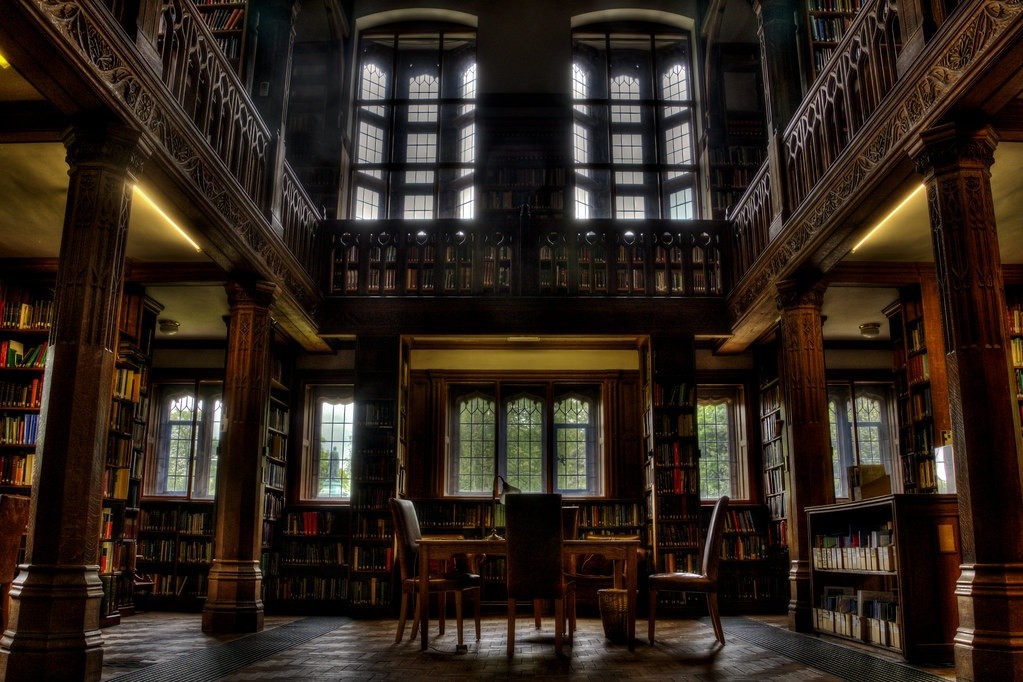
[388,495,483,651]
[503,491,580,658]
[647,496,728,647]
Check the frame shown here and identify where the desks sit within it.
[413,537,641,650]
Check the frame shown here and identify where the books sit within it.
[261,402,290,606]
[660,549,704,575]
[579,529,643,541]
[1007,302,1023,423]
[812,521,899,571]
[657,591,705,607]
[100,338,150,613]
[890,320,937,494]
[580,504,645,525]
[653,468,695,493]
[132,504,214,596]
[428,558,505,584]
[719,535,768,561]
[655,412,694,437]
[0,284,54,488]
[656,439,695,467]
[653,382,694,408]
[719,509,756,534]
[756,385,788,551]
[657,521,707,547]
[720,570,776,602]
[814,582,899,649]
[280,510,393,608]
[414,503,506,528]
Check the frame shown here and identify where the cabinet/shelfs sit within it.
[133,494,218,612]
[395,494,780,615]
[0,255,139,625]
[804,496,964,663]
[880,280,1023,497]
[343,337,412,621]
[759,371,792,555]
[280,503,341,614]
[258,336,303,615]
[129,295,170,497]
